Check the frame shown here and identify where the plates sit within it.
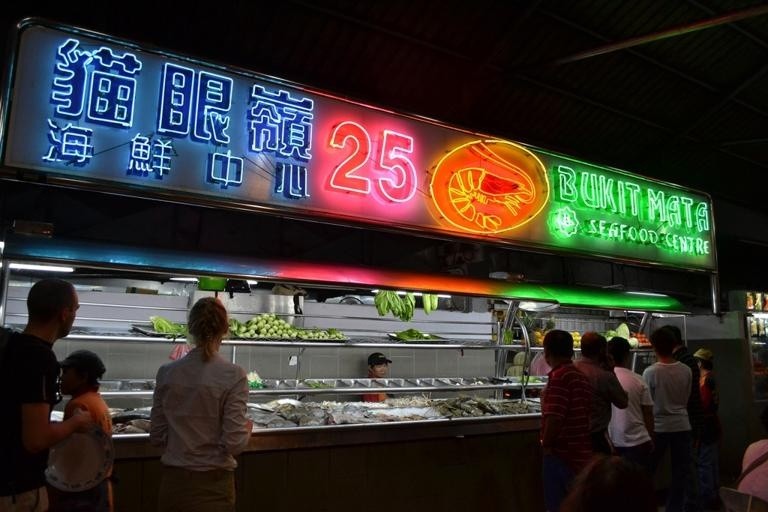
[43,424,116,494]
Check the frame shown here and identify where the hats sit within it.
[694,349,713,361]
[368,352,392,366]
[58,349,107,382]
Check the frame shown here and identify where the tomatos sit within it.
[630,331,651,347]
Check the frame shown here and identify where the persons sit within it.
[47,350,115,510]
[147,298,254,511]
[0,276,93,512]
[735,410,766,499]
[537,325,724,510]
[360,352,395,403]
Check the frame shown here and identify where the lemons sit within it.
[534,330,545,346]
[571,331,582,348]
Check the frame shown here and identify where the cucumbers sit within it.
[422,293,438,315]
